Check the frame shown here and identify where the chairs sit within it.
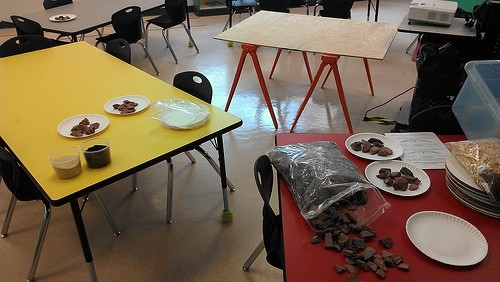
[0,0,356,282]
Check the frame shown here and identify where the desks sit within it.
[212,10,399,132]
[397,14,478,62]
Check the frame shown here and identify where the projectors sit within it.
[408,0,458,25]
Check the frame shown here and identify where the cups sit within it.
[49,145,81,179]
[80,138,112,169]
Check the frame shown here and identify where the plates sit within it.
[159,103,210,130]
[104,95,150,115]
[365,161,431,196]
[406,212,489,266]
[49,14,76,21]
[446,152,500,219]
[345,133,403,160]
[58,114,110,138]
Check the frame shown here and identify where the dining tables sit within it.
[0,41,243,282]
[274,133,500,282]
[16,0,195,47]
